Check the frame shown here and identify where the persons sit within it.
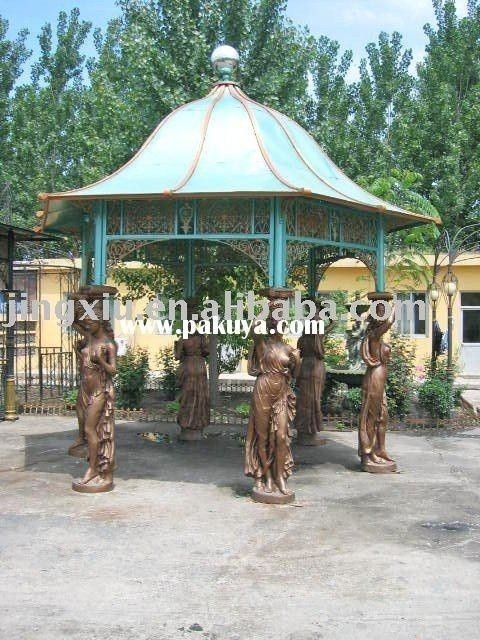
[67,336,88,450]
[297,314,336,440]
[73,307,115,484]
[174,317,210,430]
[244,316,296,494]
[358,303,396,464]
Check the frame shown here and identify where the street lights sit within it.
[427,277,441,369]
[442,264,458,385]
